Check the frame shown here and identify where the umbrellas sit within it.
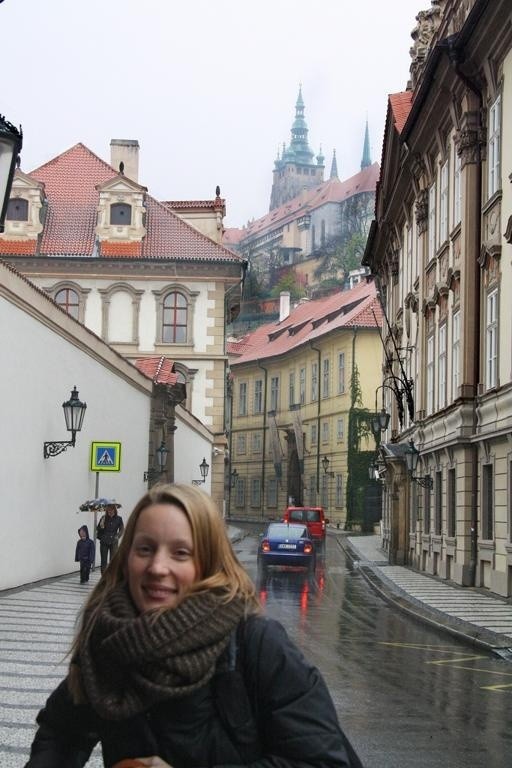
[77,497,122,517]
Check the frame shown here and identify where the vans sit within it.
[281,505,328,548]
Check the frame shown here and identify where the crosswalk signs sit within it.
[98,444,119,468]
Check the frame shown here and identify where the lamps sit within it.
[405,439,433,490]
[321,456,334,478]
[229,469,238,488]
[371,385,402,434]
[377,376,413,429]
[144,441,170,482]
[368,464,387,491]
[44,386,86,459]
[192,457,209,486]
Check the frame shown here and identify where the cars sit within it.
[257,522,318,574]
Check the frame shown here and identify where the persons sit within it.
[95,504,124,576]
[75,522,96,584]
[22,481,366,768]
